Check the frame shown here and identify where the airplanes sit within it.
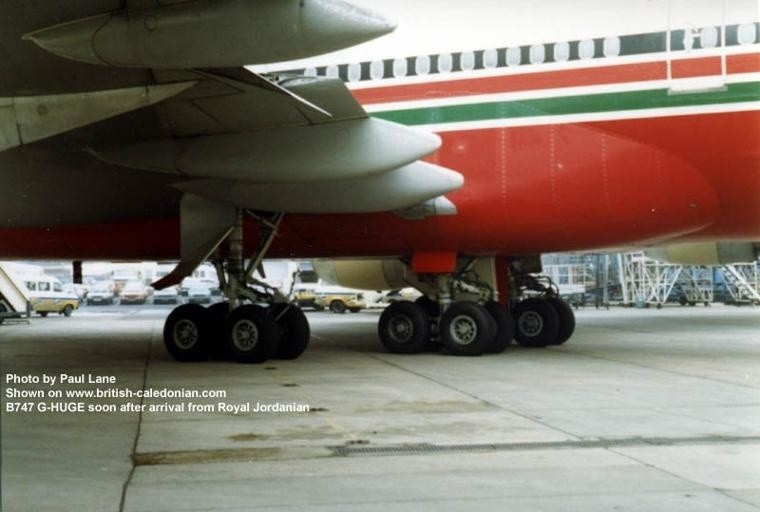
[0,2,760,362]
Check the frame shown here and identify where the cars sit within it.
[62,272,220,304]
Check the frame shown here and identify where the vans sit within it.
[0,262,78,317]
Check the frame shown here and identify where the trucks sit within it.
[287,266,384,315]
